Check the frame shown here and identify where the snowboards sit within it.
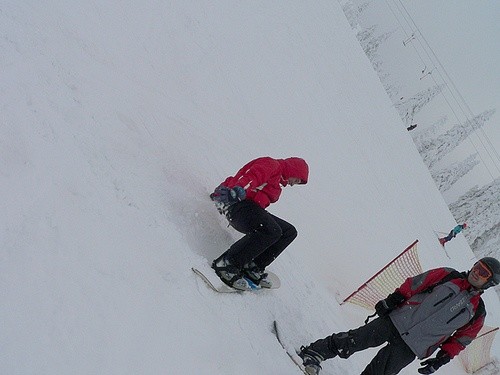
[192,258,280,293]
[274,320,314,375]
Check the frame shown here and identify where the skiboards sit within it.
[436,232,452,259]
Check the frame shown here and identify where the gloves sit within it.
[374,291,406,316]
[418,348,453,375]
[210,186,246,210]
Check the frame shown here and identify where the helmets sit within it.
[473,256,500,290]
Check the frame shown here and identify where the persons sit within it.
[299,257,500,375]
[439,222,468,247]
[209,157,308,291]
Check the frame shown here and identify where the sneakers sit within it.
[302,349,323,374]
[242,262,272,289]
[215,260,250,291]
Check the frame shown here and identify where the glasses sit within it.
[473,261,491,281]
